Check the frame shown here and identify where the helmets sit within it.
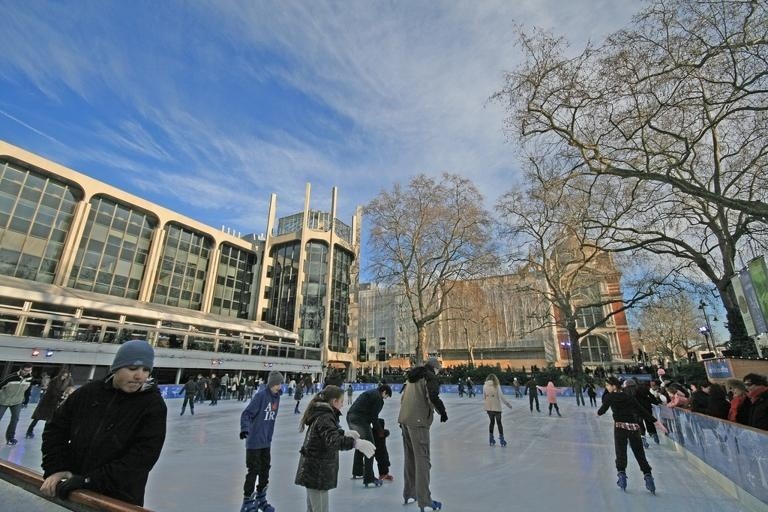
[622,378,637,389]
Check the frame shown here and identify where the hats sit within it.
[109,338,156,374]
[427,357,442,370]
[267,369,286,390]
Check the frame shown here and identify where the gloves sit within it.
[344,429,361,441]
[239,430,250,440]
[352,438,377,460]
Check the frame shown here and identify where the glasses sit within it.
[744,382,756,388]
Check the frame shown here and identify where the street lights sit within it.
[601,352,608,377]
[698,298,719,357]
[561,340,575,392]
[637,327,646,365]
[698,326,712,355]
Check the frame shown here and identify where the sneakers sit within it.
[179,391,321,416]
[529,409,563,419]
[403,494,417,500]
[421,499,442,508]
[347,401,353,406]
[352,473,384,484]
[5,437,18,444]
[515,395,524,400]
[577,403,598,409]
[460,393,477,400]
[26,431,35,438]
[378,474,394,480]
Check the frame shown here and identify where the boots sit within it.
[239,490,259,512]
[651,433,660,444]
[616,470,628,488]
[498,433,507,446]
[641,435,650,448]
[643,472,656,491]
[489,432,497,444]
[255,485,276,512]
